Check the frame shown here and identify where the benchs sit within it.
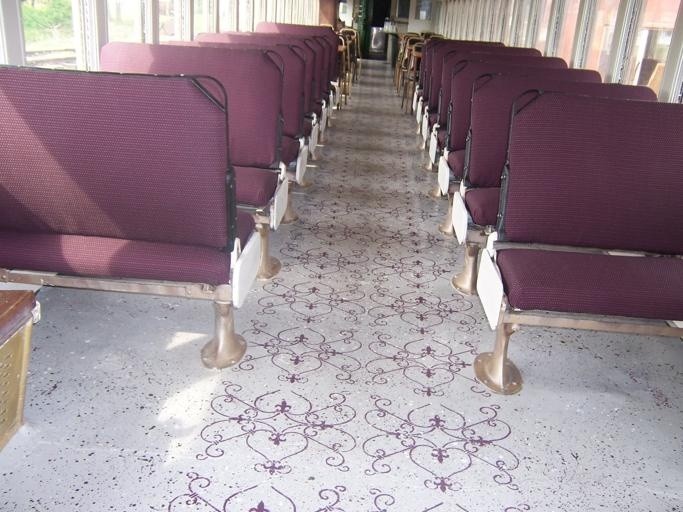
[411,41,683,394]
[0,20,341,366]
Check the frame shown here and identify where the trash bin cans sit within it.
[371,28,397,62]
[0,291,36,450]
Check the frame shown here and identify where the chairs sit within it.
[319,23,358,109]
[395,31,444,114]
